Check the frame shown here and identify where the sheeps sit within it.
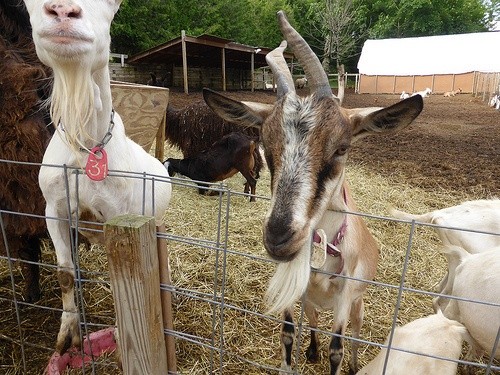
[352,294,483,375]
[434,243,500,364]
[264,80,277,89]
[157,140,259,195]
[18,0,172,358]
[399,91,409,100]
[200,7,423,375]
[384,198,499,295]
[0,4,91,303]
[295,78,307,89]
[162,99,262,202]
[411,88,463,98]
